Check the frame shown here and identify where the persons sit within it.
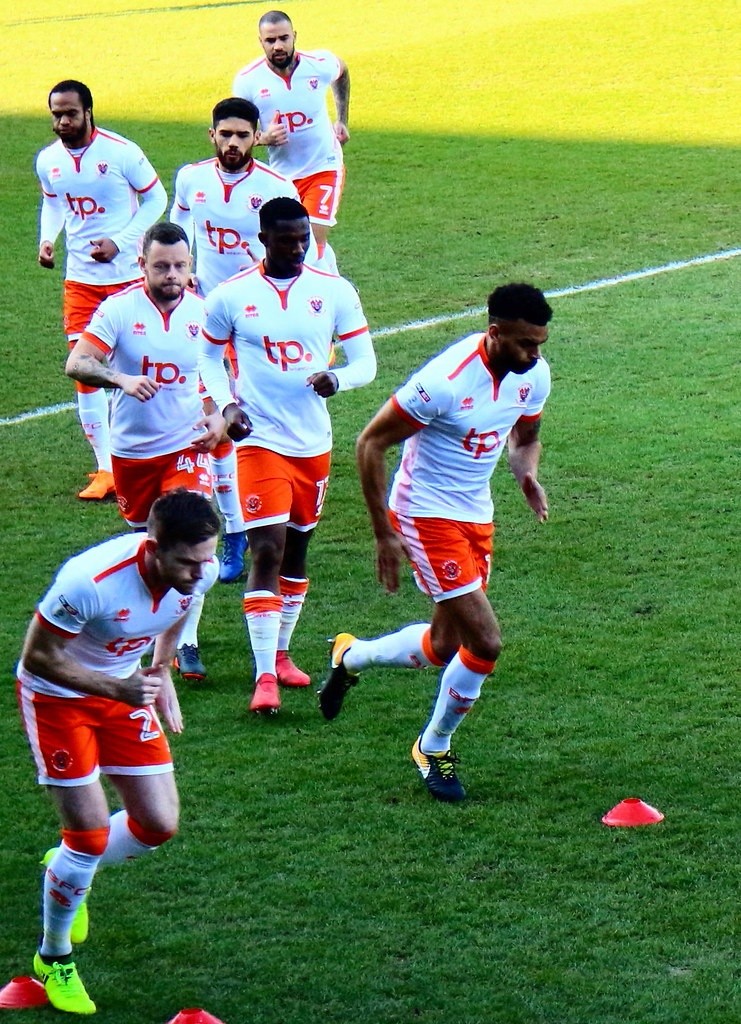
[13,481,225,1018]
[197,195,378,715]
[166,95,320,591]
[233,7,351,373]
[35,77,169,503]
[61,220,234,684]
[316,281,559,805]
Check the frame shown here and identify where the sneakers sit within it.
[248,673,280,714]
[317,631,360,721]
[30,948,97,1014]
[217,529,251,582]
[173,642,208,681]
[276,649,312,686]
[37,845,89,943]
[78,469,115,500]
[411,734,466,800]
